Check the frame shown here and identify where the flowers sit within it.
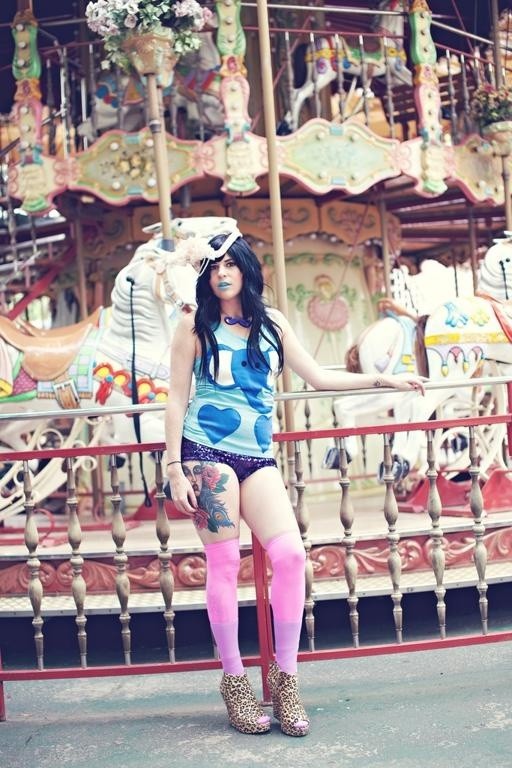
[85,0,212,70]
[469,81,511,125]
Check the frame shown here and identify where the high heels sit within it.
[217,670,276,736]
[260,658,312,740]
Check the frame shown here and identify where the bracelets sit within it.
[164,459,181,466]
[372,373,383,388]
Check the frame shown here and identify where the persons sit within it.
[182,459,204,495]
[163,232,430,736]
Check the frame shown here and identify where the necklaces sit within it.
[221,309,253,330]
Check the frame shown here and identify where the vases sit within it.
[118,33,181,76]
[480,122,511,157]
